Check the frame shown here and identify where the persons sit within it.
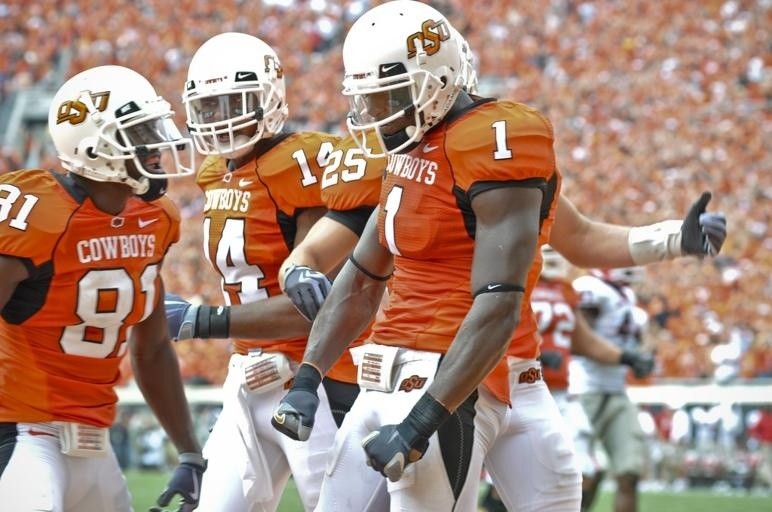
[0,1,771,387]
[164,33,394,511]
[0,66,208,512]
[271,1,728,512]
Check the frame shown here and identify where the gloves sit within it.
[278,263,334,318]
[680,190,727,257]
[360,421,429,482]
[144,451,207,512]
[540,352,564,370]
[272,384,320,443]
[160,292,202,343]
[631,350,655,377]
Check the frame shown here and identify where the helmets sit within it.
[339,0,478,159]
[46,63,196,200]
[540,242,573,281]
[179,31,290,157]
[593,263,647,284]
